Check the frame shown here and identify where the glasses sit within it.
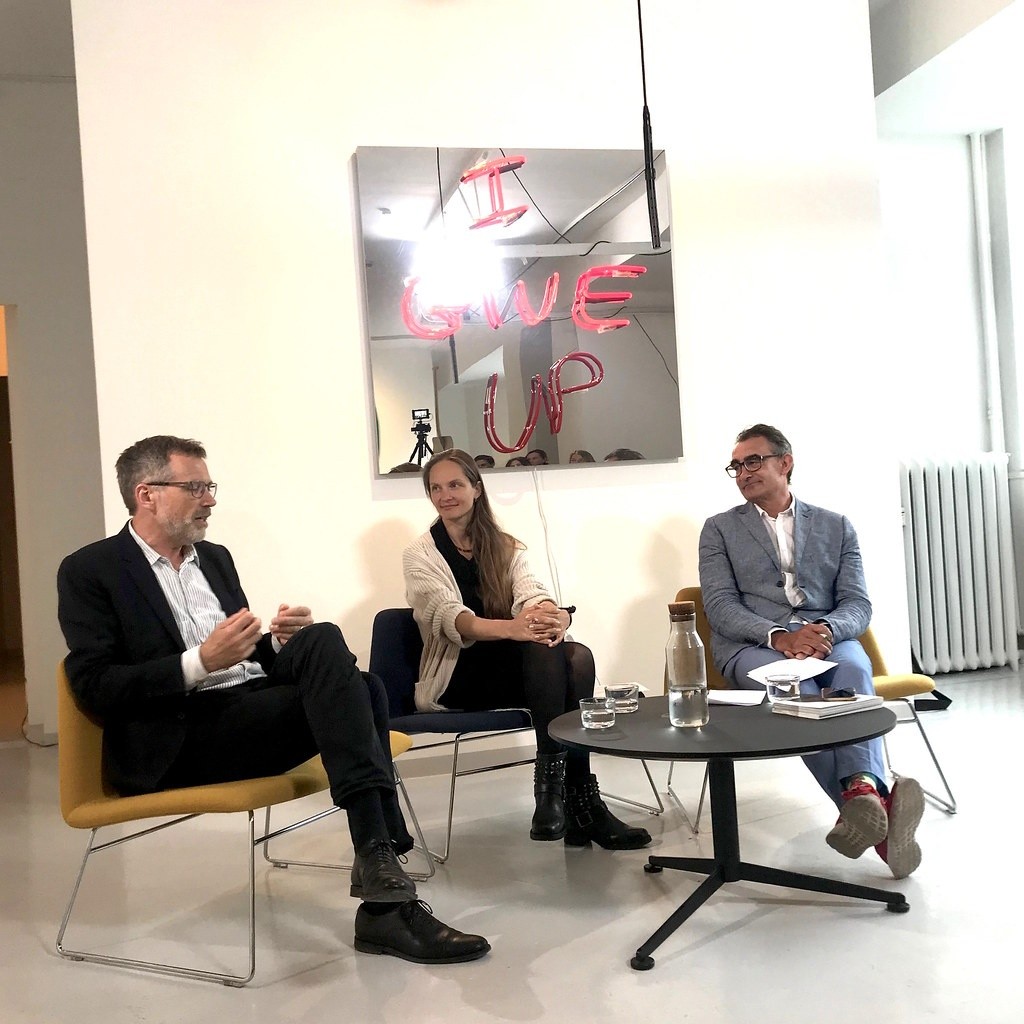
[725,454,779,478]
[146,480,217,498]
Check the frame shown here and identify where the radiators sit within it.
[900,451,1024,675]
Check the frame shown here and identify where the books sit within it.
[772,693,883,720]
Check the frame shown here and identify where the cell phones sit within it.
[820,686,857,701]
[412,409,429,419]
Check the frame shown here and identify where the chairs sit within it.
[664,588,957,835]
[57,660,435,989]
[369,609,664,864]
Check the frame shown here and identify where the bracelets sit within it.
[557,606,576,631]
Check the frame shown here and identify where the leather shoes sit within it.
[350,835,418,902]
[354,900,491,964]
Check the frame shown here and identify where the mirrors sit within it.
[355,146,683,476]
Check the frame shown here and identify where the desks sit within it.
[548,696,910,969]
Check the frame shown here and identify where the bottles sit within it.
[665,603,710,727]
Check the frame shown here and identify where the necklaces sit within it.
[455,545,473,552]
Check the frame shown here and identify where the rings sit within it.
[300,625,304,629]
[531,617,539,624]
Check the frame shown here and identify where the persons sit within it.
[402,448,653,851]
[388,449,647,473]
[56,435,493,964]
[698,423,925,879]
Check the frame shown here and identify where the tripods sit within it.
[409,421,434,466]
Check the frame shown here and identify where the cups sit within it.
[603,683,639,713]
[765,675,801,703]
[580,697,617,729]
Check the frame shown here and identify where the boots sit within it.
[530,750,568,841]
[564,774,652,850]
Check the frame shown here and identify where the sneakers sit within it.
[875,778,925,879]
[826,780,888,859]
